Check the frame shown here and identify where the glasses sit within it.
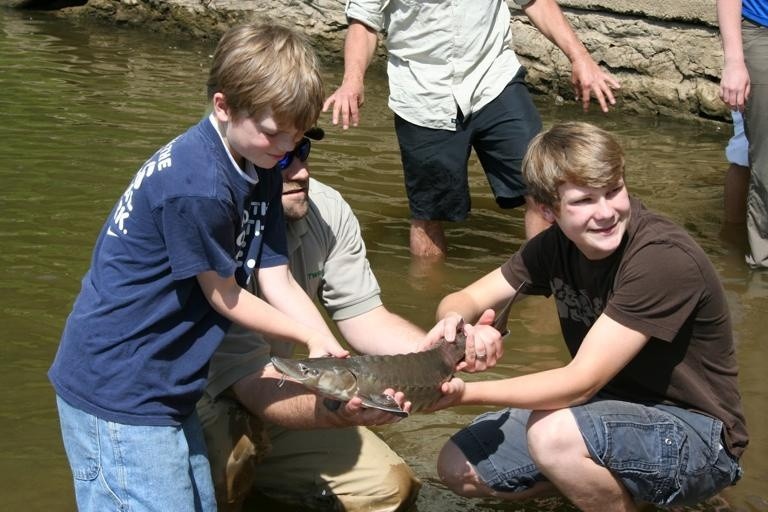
[273,139,309,171]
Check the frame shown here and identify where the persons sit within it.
[195,125,504,511]
[44,24,327,512]
[715,0,768,269]
[320,0,622,259]
[420,120,750,512]
[724,107,750,224]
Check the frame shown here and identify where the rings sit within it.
[476,355,486,359]
